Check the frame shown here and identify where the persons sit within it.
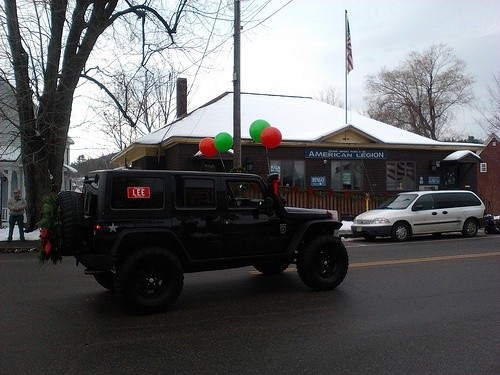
[3,188,28,243]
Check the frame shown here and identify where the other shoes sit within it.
[7,240,12,242]
[21,238,25,241]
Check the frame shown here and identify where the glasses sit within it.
[13,192,18,194]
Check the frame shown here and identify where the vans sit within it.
[350,191,487,241]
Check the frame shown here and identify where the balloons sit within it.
[199,137,220,158]
[213,132,234,153]
[249,119,271,146]
[260,126,283,150]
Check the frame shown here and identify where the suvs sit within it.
[54,169,349,314]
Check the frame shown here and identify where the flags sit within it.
[344,10,353,74]
